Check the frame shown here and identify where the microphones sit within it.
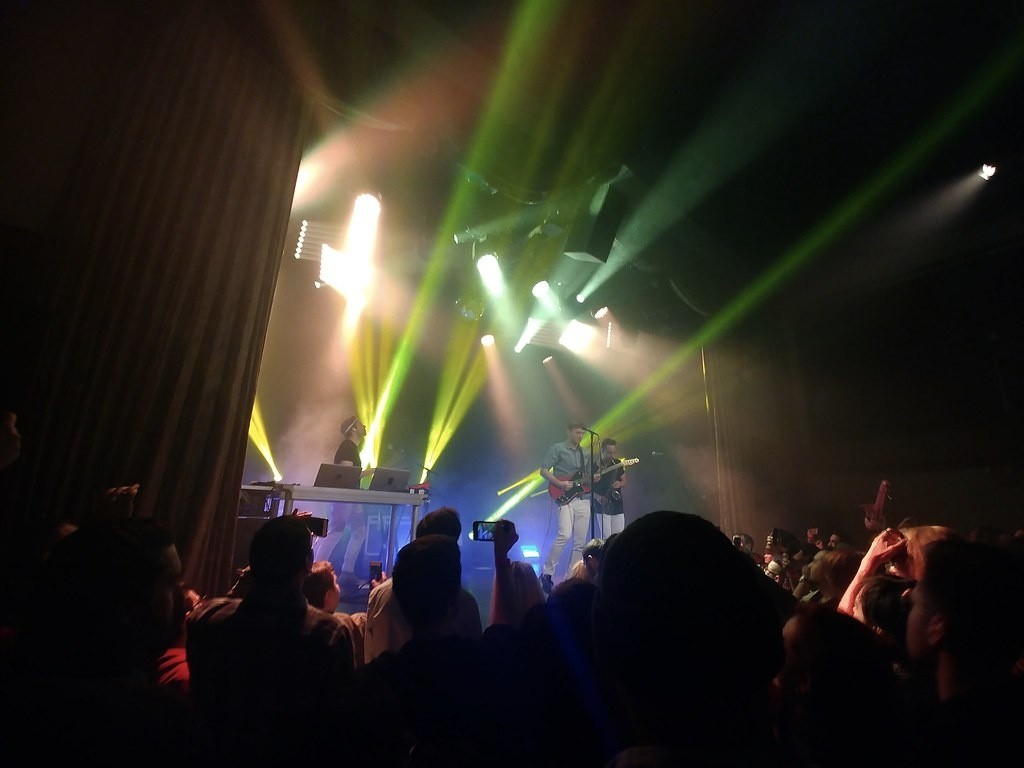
[580,424,598,437]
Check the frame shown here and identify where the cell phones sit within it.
[368,560,383,589]
[471,520,505,543]
[733,537,741,549]
[303,515,329,539]
[807,527,818,536]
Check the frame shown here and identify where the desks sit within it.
[281,485,429,579]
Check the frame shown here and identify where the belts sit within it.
[576,493,590,499]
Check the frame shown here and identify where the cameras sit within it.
[772,528,779,543]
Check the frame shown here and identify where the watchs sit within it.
[798,575,809,582]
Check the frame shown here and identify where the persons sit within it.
[0,412,1023,768]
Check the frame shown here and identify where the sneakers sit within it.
[539,573,553,596]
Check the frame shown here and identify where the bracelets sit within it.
[494,558,511,568]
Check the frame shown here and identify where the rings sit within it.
[885,529,892,535]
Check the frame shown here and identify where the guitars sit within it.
[548,457,640,505]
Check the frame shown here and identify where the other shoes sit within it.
[338,572,367,587]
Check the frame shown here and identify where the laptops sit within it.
[367,467,412,492]
[312,462,362,489]
